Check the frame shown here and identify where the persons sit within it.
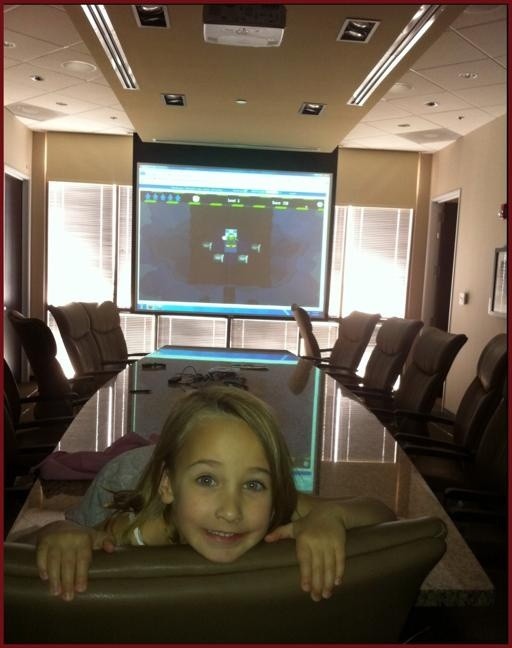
[37,382,398,603]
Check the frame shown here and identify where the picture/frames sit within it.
[488,247,507,318]
